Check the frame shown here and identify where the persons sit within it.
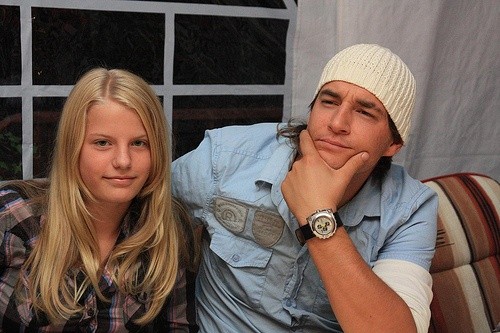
[164,43,437,333]
[0,66,198,333]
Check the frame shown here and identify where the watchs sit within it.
[292,208,344,248]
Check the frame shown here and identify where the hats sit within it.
[313,42,416,147]
